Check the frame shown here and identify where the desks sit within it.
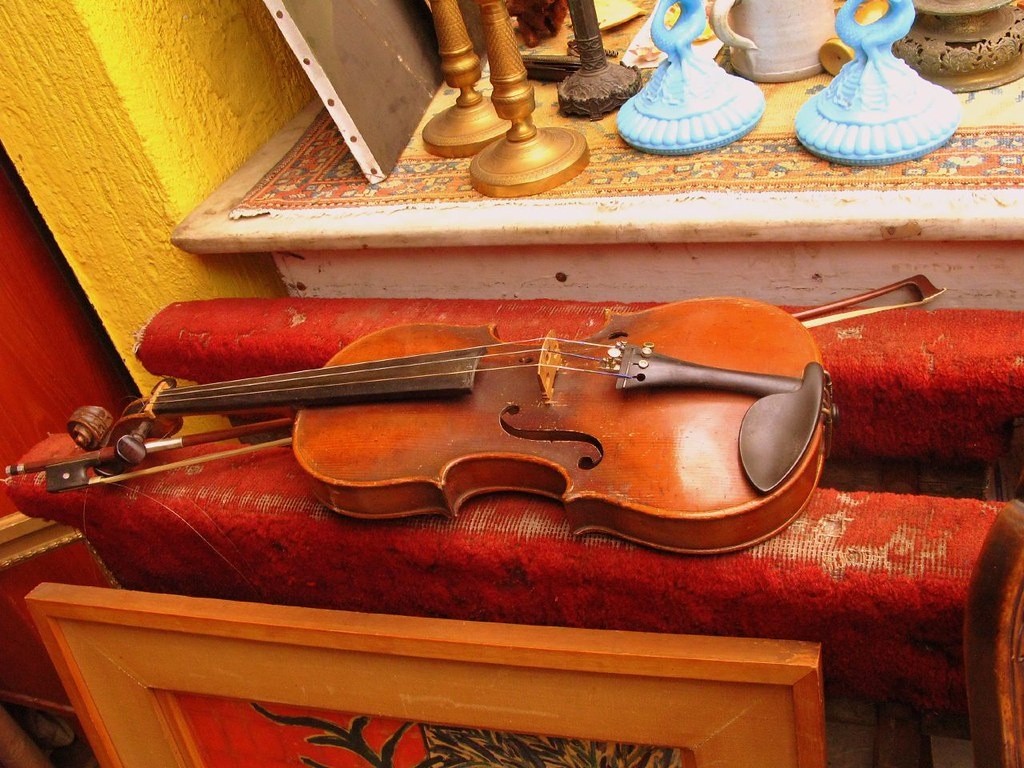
[170,0,1024,322]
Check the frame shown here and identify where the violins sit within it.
[65,295,840,557]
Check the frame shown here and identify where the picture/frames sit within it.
[21,580,830,768]
